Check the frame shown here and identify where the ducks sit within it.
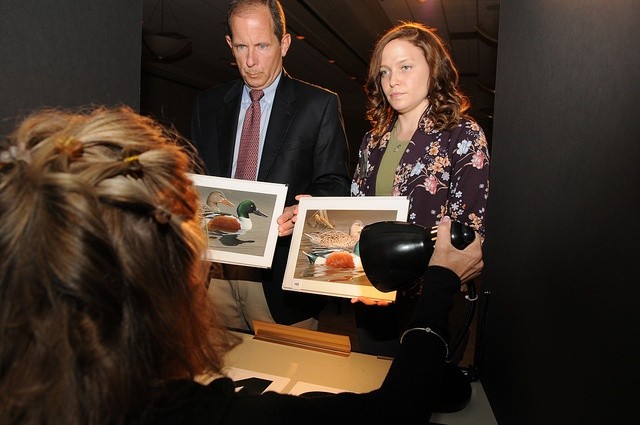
[200,190,236,212]
[303,219,365,253]
[199,200,268,237]
[209,236,256,246]
[302,241,363,268]
[299,266,366,283]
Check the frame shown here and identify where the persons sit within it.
[347,22,490,365]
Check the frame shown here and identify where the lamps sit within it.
[358,221,479,413]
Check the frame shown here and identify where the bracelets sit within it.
[400,327,449,358]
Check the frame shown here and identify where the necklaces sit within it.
[393,120,416,151]
[0,104,482,424]
[198,0,349,332]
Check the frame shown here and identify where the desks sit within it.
[190,327,498,425]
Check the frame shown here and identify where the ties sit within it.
[234,90,265,181]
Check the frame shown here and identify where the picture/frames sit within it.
[182,172,290,269]
[281,197,409,304]
